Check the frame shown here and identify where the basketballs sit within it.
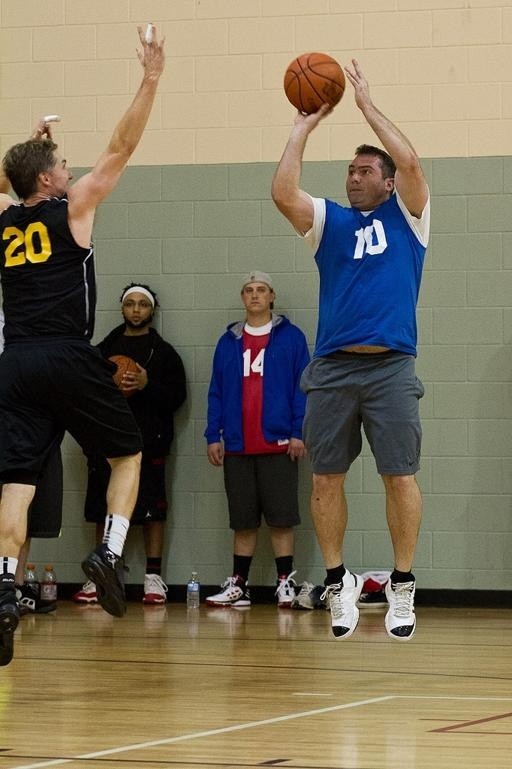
[285,53,346,113]
[107,356,140,397]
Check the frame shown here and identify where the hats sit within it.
[239,268,277,311]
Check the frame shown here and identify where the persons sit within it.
[199,271,311,609]
[0,23,167,665]
[271,59,432,643]
[0,277,64,617]
[71,282,187,604]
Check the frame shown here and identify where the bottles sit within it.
[40,564,60,609]
[186,611,200,642]
[23,563,39,593]
[185,573,199,611]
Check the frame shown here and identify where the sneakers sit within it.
[277,574,296,608]
[291,581,322,612]
[142,573,169,605]
[384,576,418,641]
[82,544,127,618]
[203,574,251,608]
[74,581,98,603]
[357,591,390,609]
[0,573,21,667]
[323,569,365,641]
[14,584,59,616]
